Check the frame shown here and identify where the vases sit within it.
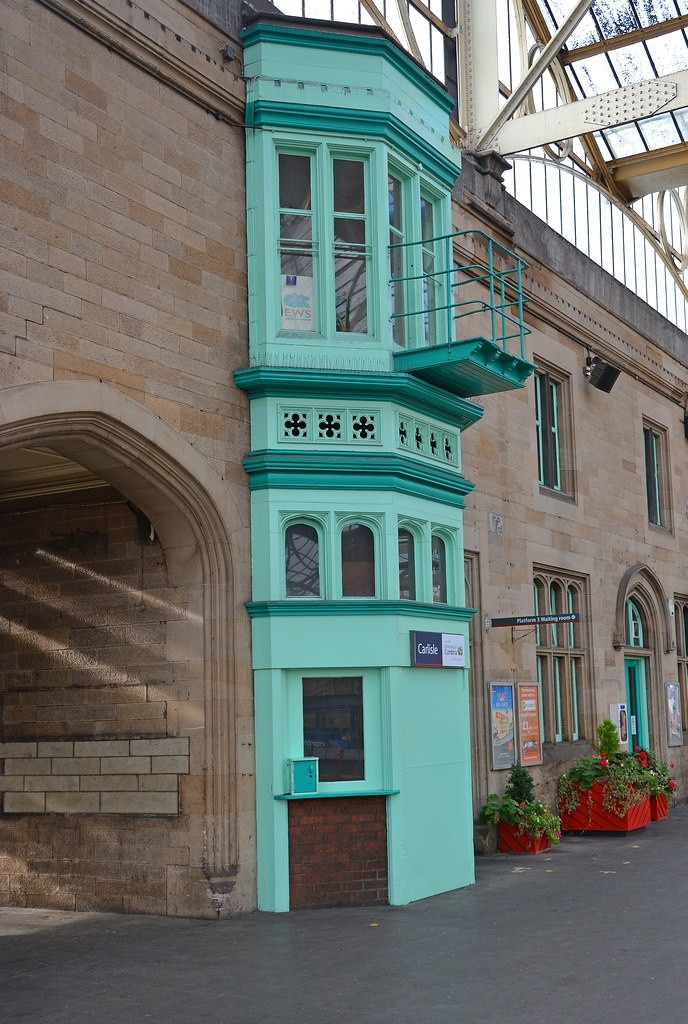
[560,777,653,834]
[497,820,553,855]
[649,793,668,822]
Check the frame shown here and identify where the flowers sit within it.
[560,746,680,818]
[481,792,563,843]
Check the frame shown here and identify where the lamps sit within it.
[583,347,622,393]
